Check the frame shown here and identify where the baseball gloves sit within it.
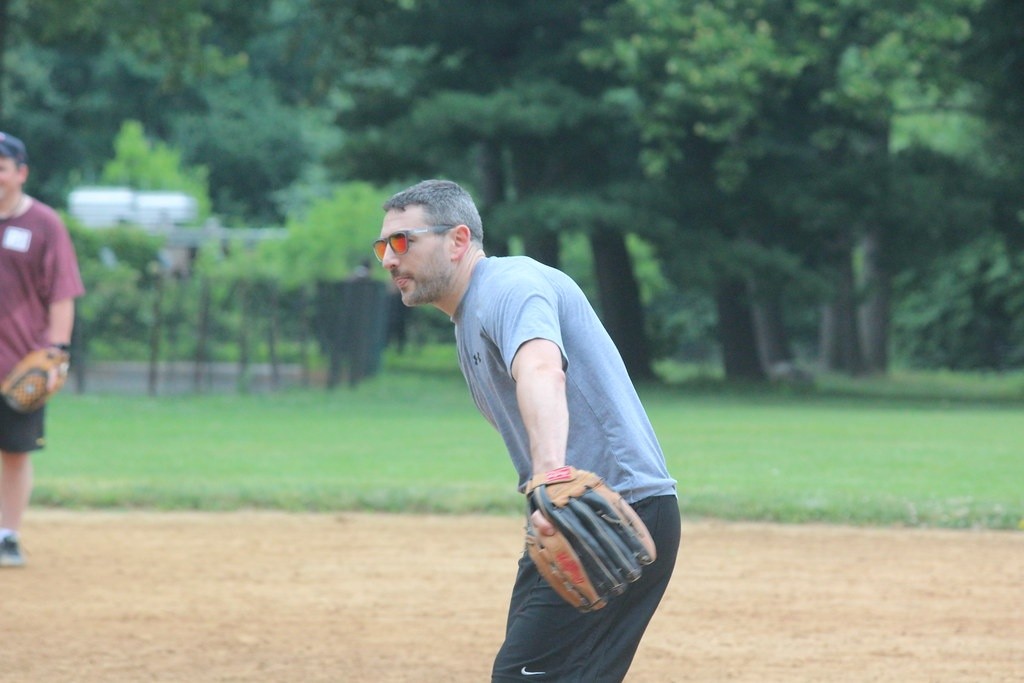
[1,342,73,415]
[518,463,658,616]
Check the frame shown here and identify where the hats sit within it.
[0,130,26,162]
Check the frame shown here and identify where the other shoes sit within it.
[0,534,23,567]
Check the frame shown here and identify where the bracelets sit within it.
[50,344,70,349]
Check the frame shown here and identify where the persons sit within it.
[373,181,681,683]
[0,130,85,568]
[342,260,408,387]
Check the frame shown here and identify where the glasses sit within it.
[372,225,454,262]
